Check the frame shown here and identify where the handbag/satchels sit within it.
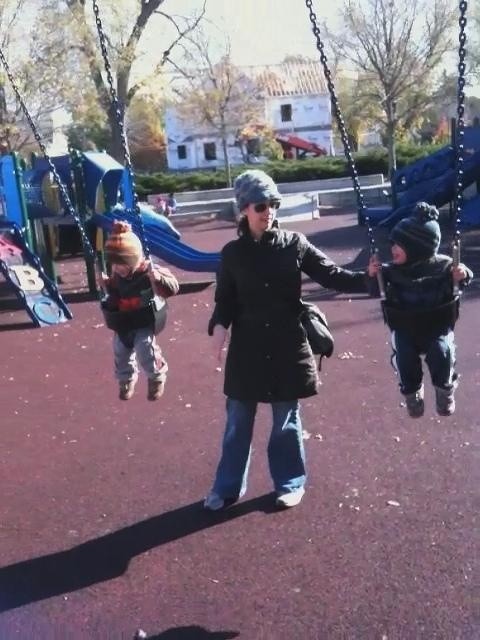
[302,298,337,357]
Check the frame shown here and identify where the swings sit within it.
[301,1,467,348]
[1,0,167,344]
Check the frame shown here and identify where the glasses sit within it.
[254,200,281,215]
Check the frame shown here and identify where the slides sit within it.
[357,124,479,227]
[93,208,222,274]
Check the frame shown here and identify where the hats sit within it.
[387,203,443,256]
[231,167,284,210]
[103,216,144,271]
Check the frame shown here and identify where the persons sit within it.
[203,169,367,511]
[168,192,177,215]
[97,227,179,400]
[368,204,473,417]
[156,198,167,214]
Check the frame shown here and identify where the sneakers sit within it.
[400,384,428,419]
[116,373,140,402]
[203,487,225,512]
[145,375,166,403]
[436,383,457,420]
[272,484,306,509]
